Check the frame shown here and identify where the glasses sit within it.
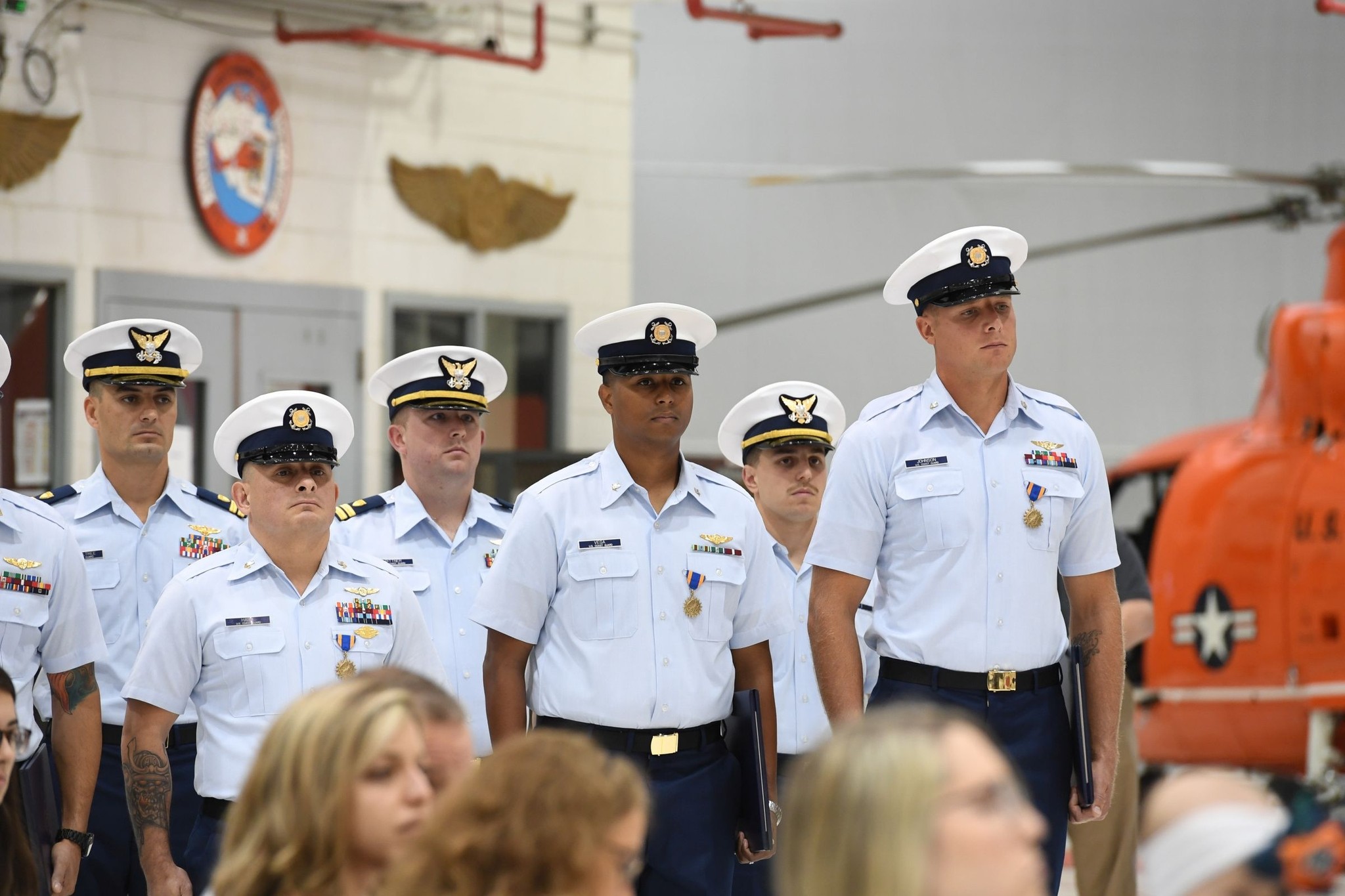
[0,729,33,755]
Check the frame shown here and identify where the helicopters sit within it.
[681,134,1344,895]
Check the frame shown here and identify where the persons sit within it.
[115,389,445,896]
[0,338,106,895]
[717,380,888,808]
[805,224,1128,896]
[761,700,1054,896]
[416,727,654,896]
[31,314,253,896]
[195,670,438,896]
[298,345,531,767]
[355,663,468,795]
[469,303,784,896]
[0,668,52,896]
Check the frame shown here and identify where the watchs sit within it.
[765,798,782,827]
[55,829,95,858]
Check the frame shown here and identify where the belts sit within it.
[534,716,721,755]
[878,657,1059,693]
[774,758,814,782]
[101,723,197,749]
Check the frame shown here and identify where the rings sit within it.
[749,861,754,865]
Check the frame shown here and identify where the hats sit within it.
[0,334,12,389]
[63,319,203,388]
[213,390,355,480]
[717,381,846,468]
[882,226,1028,310]
[367,346,509,414]
[574,303,717,376]
